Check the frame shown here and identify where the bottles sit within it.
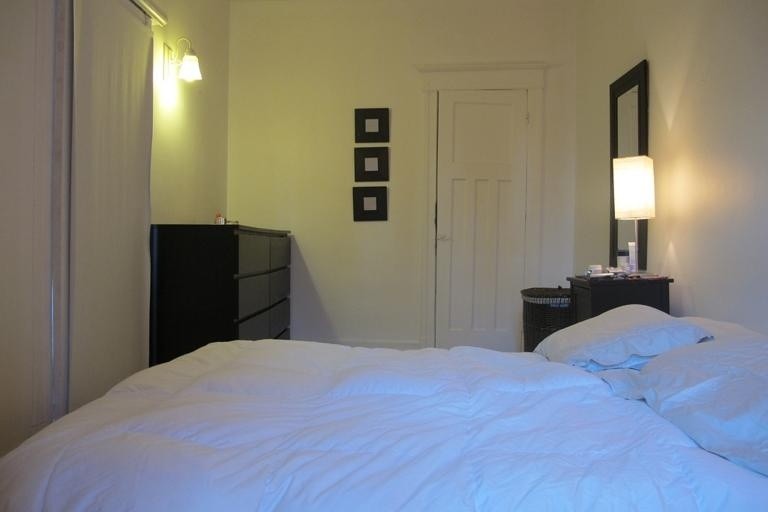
[617,251,628,269]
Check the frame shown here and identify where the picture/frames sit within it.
[353,107,390,223]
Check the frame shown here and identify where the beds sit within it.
[1,316,767,512]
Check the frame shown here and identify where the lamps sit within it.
[163,37,203,80]
[613,155,655,277]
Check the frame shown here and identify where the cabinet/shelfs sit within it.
[149,224,291,367]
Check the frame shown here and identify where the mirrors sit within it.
[609,59,650,271]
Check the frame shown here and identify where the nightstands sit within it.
[566,273,674,325]
